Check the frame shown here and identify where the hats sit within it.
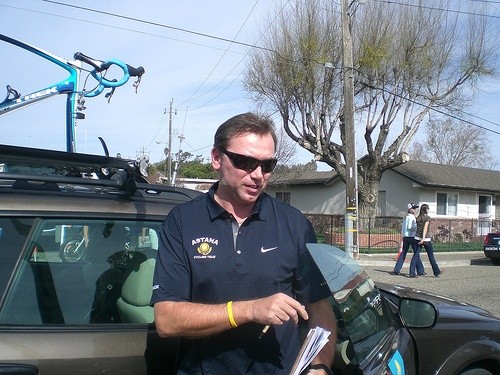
[408,202,419,210]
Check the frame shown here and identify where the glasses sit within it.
[219,146,277,173]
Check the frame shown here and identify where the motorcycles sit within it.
[58,225,135,264]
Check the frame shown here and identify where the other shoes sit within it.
[409,275,419,278]
[392,268,402,275]
[418,272,428,274]
[435,270,444,277]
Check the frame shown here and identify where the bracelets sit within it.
[311,363,331,375]
[227,300,239,328]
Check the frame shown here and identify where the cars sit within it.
[484,231,500,267]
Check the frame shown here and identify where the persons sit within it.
[409,204,444,278]
[89,250,146,323]
[392,203,426,275]
[150,112,338,375]
[84,225,148,263]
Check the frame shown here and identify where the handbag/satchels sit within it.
[396,239,408,262]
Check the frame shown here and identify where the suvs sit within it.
[0,145,500,375]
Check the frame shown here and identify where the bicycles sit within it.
[0,34,146,155]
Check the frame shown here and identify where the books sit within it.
[290,326,331,375]
